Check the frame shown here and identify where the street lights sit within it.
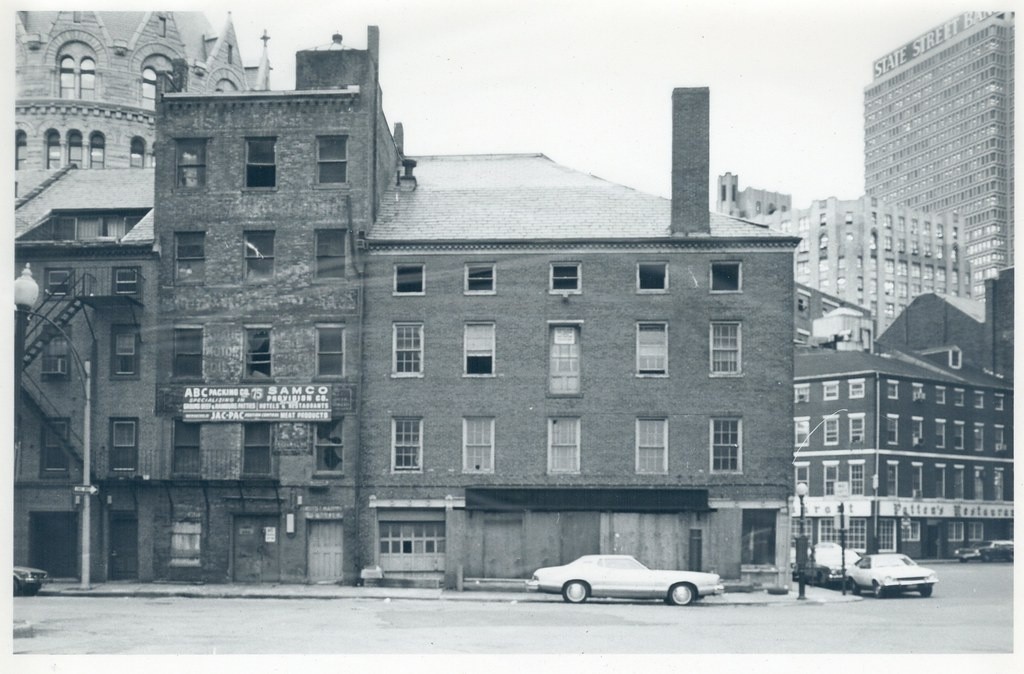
[797,483,808,538]
[14,262,39,443]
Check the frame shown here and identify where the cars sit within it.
[845,554,940,599]
[793,543,861,586]
[954,541,1014,562]
[528,554,723,606]
[14,566,48,596]
